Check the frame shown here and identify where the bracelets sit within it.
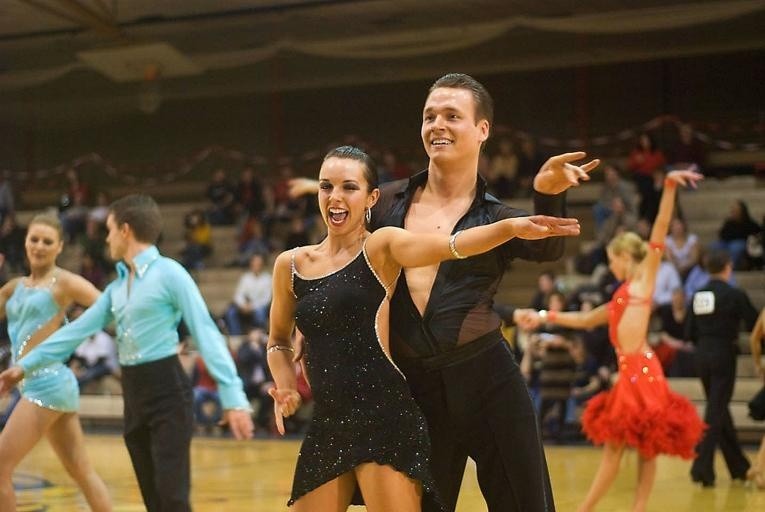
[450,229,468,261]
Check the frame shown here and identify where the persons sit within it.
[747,304,765,492]
[483,121,765,442]
[361,74,602,512]
[528,164,704,512]
[0,196,255,511]
[266,146,583,511]
[670,248,757,484]
[1,135,410,434]
[0,212,115,512]
